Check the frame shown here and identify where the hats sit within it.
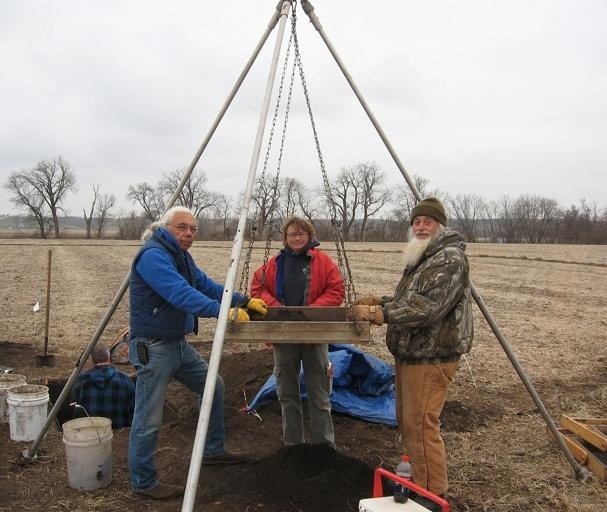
[410,198,446,226]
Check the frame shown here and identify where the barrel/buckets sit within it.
[62,405,114,492]
[7,384,49,442]
[0,373,28,426]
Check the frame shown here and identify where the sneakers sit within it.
[139,483,184,500]
[202,451,250,465]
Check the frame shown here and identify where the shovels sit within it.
[36,249,55,368]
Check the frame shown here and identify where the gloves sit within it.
[347,305,385,326]
[228,307,251,321]
[354,294,382,305]
[247,298,268,318]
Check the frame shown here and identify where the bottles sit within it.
[393,454,411,504]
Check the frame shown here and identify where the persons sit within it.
[126,205,268,502]
[350,197,474,512]
[69,342,135,429]
[248,215,347,447]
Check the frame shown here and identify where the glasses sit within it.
[286,231,309,239]
[166,223,200,234]
